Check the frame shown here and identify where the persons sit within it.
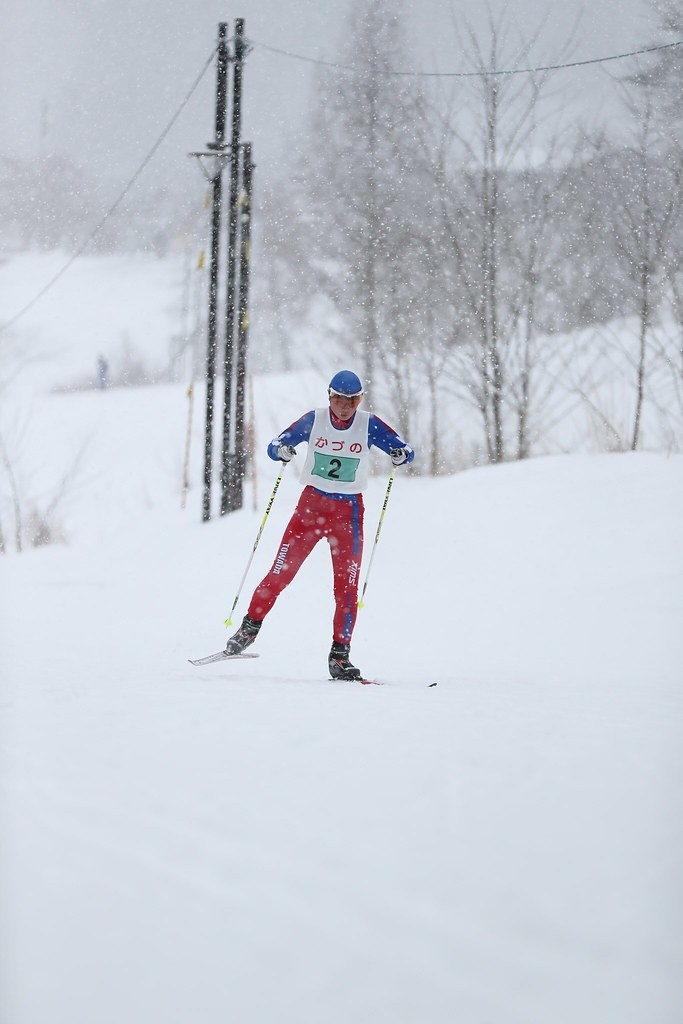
[226,369,416,677]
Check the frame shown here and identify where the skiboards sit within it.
[187,649,438,690]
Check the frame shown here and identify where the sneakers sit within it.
[226,614,262,652]
[328,643,360,678]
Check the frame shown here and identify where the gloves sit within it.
[391,448,410,466]
[277,444,297,462]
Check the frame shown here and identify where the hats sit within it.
[328,370,363,398]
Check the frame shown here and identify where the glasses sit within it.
[329,387,364,408]
